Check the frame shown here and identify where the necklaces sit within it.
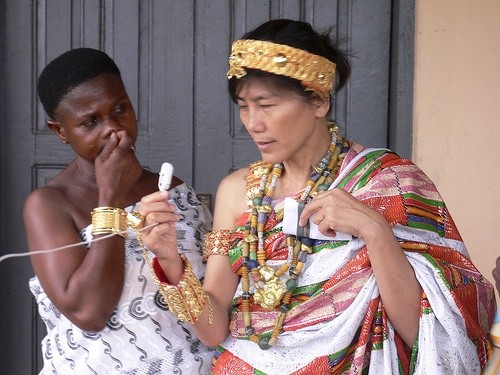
[241,121,348,350]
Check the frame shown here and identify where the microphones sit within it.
[158,162,174,192]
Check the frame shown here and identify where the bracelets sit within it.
[90,207,129,239]
[135,231,213,325]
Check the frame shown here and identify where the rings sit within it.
[127,211,145,228]
[130,145,136,152]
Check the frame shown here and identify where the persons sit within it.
[492,256,500,298]
[127,18,497,375]
[22,48,213,375]
